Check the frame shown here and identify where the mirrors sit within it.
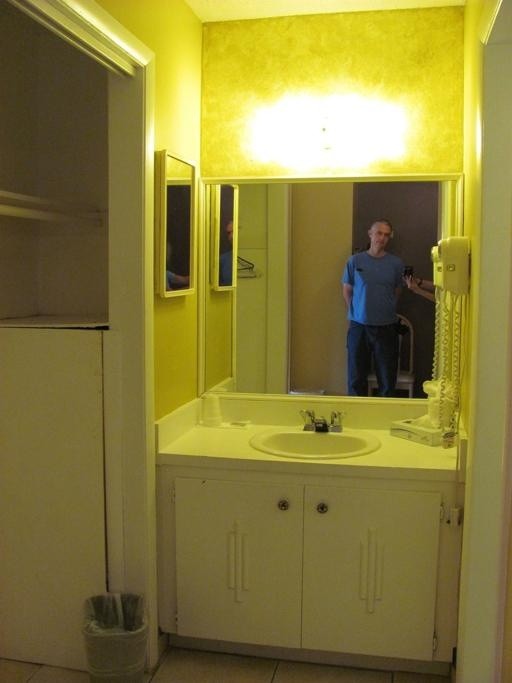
[197,173,466,405]
[158,149,198,299]
[213,184,240,291]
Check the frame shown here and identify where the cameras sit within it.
[402,267,413,280]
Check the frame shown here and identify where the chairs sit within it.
[366,313,417,399]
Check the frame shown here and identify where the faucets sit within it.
[312,415,329,432]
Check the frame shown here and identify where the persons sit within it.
[400,270,436,304]
[219,218,233,286]
[341,216,405,398]
[166,241,188,289]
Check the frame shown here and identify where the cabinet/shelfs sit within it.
[0,311,108,673]
[169,476,445,662]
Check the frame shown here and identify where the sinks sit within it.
[250,428,381,459]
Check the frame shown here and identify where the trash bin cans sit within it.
[81,593,150,683]
[289,390,327,395]
